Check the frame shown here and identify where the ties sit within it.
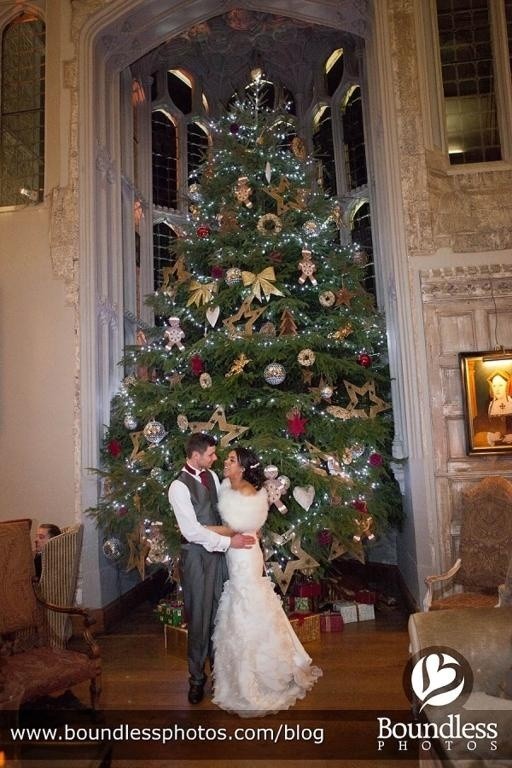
[183,462,209,489]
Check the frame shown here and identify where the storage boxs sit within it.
[276,560,376,647]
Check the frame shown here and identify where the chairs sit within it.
[1,515,108,764]
[407,609,512,766]
[420,470,512,613]
[34,516,84,648]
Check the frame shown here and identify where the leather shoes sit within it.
[187,673,207,705]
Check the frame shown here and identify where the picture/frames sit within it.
[458,346,512,456]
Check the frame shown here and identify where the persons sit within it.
[34,524,62,580]
[168,433,263,704]
[473,369,512,447]
[174,447,323,719]
